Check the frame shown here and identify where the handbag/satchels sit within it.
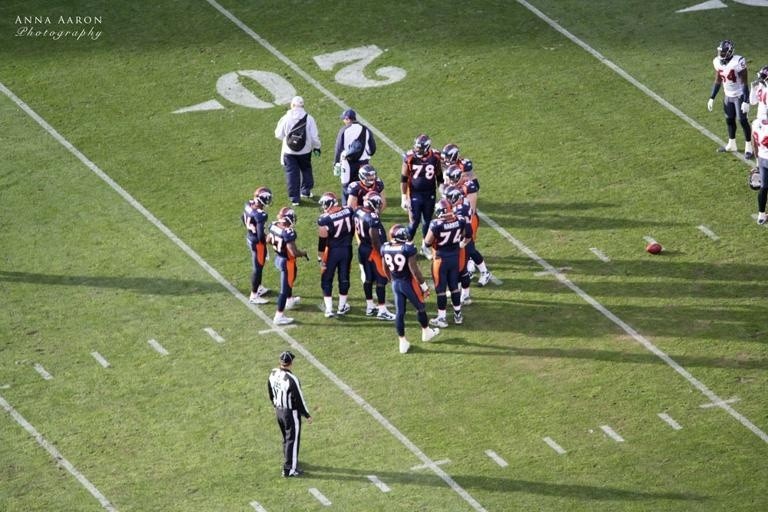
[286,114,308,151]
[342,138,364,162]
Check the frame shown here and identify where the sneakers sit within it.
[292,470,302,477]
[247,285,395,325]
[717,146,737,152]
[300,193,314,198]
[399,260,490,354]
[744,147,754,159]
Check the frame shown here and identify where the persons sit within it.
[239,186,273,304]
[266,206,309,326]
[267,351,314,480]
[348,164,385,245]
[352,190,396,321]
[332,107,378,206]
[399,133,444,261]
[380,222,440,354]
[445,185,492,306]
[317,192,355,318]
[450,160,480,280]
[441,143,472,185]
[274,96,323,206]
[706,39,768,226]
[425,198,463,328]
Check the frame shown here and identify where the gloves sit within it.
[741,101,750,114]
[707,98,714,112]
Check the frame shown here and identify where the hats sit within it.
[292,96,304,106]
[340,108,356,121]
[279,351,295,366]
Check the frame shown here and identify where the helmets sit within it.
[717,39,735,64]
[253,185,273,208]
[318,164,411,244]
[757,67,768,86]
[277,205,297,226]
[414,133,462,217]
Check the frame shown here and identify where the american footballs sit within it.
[646,243,661,252]
